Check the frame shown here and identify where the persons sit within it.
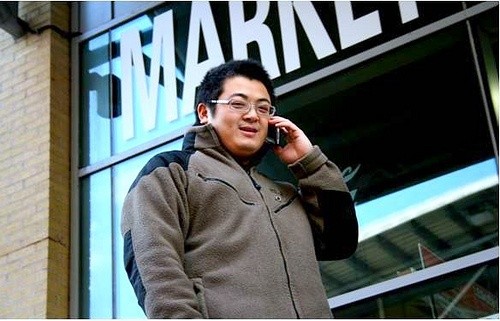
[121,60,358,318]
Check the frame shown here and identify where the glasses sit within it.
[196,99,276,117]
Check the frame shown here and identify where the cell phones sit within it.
[264,125,284,146]
